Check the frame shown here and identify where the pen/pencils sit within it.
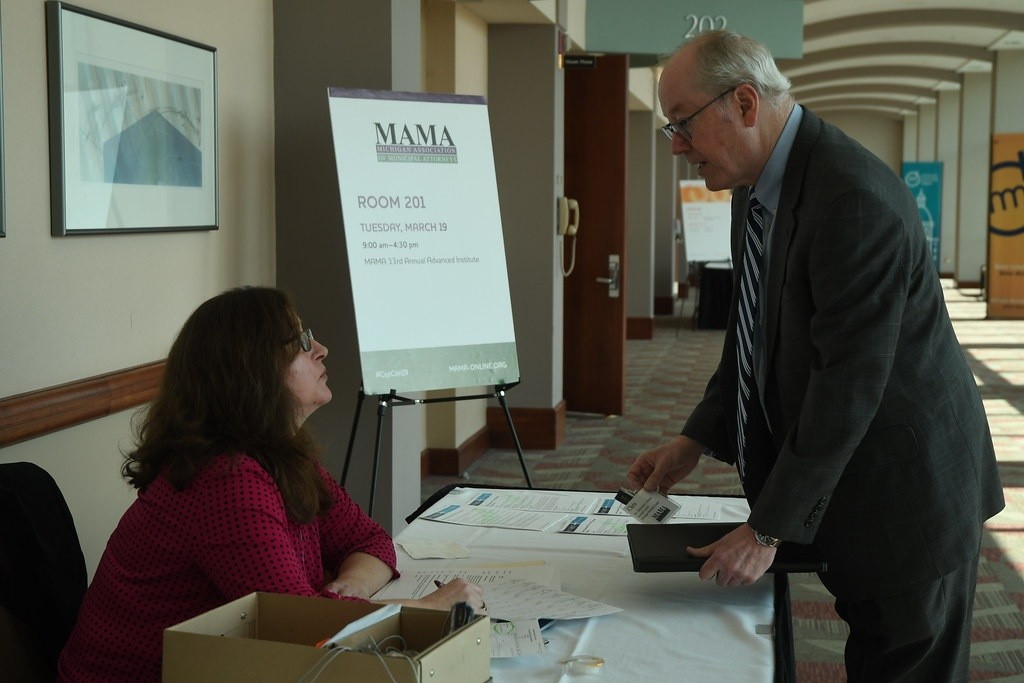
[434,580,444,588]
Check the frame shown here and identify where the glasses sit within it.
[285,328,314,352]
[661,88,736,143]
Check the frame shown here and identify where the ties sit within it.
[737,187,763,481]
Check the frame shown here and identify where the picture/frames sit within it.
[44,0,220,237]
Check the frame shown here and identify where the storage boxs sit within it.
[161,591,493,683]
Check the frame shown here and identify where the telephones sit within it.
[558,197,579,234]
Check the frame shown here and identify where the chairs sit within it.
[0,462,89,683]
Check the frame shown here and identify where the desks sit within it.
[370,482,797,682]
[697,263,734,328]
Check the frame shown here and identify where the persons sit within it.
[627,30,1007,681]
[52,287,487,683]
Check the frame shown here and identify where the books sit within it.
[625,523,820,573]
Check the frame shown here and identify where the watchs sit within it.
[747,522,782,547]
